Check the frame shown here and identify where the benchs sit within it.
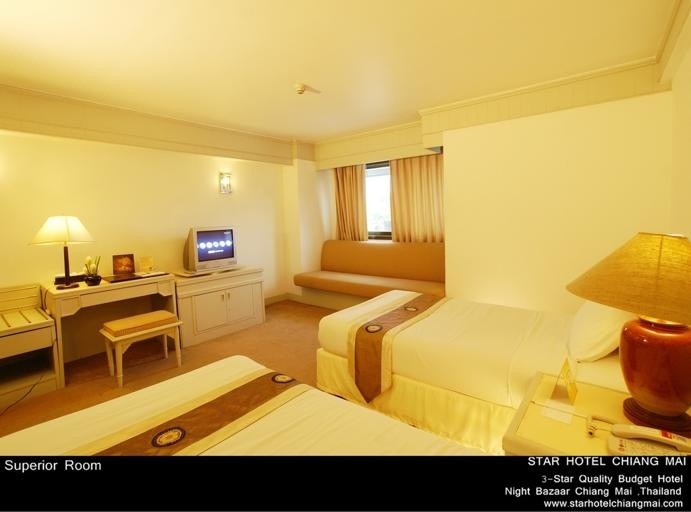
[294,242,445,310]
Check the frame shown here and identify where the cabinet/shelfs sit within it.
[174,265,266,349]
[0,283,60,408]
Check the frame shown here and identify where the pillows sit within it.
[567,298,639,363]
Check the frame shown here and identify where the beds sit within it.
[315,286,691,449]
[0,354,490,458]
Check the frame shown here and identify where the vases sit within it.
[85,274,101,286]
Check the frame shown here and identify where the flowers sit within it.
[84,256,102,274]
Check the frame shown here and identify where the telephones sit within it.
[608,426,690,456]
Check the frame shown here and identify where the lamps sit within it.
[28,216,93,290]
[567,230,691,438]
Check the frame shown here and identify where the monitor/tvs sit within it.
[183,224,238,271]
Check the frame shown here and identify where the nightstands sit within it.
[502,373,691,458]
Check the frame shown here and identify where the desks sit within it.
[40,270,178,385]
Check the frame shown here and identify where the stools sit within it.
[100,309,183,388]
